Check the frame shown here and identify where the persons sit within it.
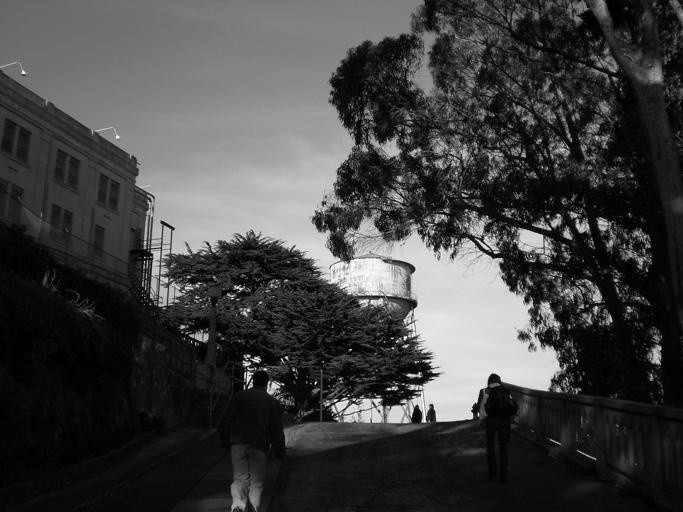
[214,369,287,511]
[410,403,423,424]
[470,372,518,485]
[426,403,437,423]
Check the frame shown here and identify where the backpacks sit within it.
[485,385,519,419]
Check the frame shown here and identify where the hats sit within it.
[487,374,502,384]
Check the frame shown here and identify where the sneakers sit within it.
[488,475,509,482]
